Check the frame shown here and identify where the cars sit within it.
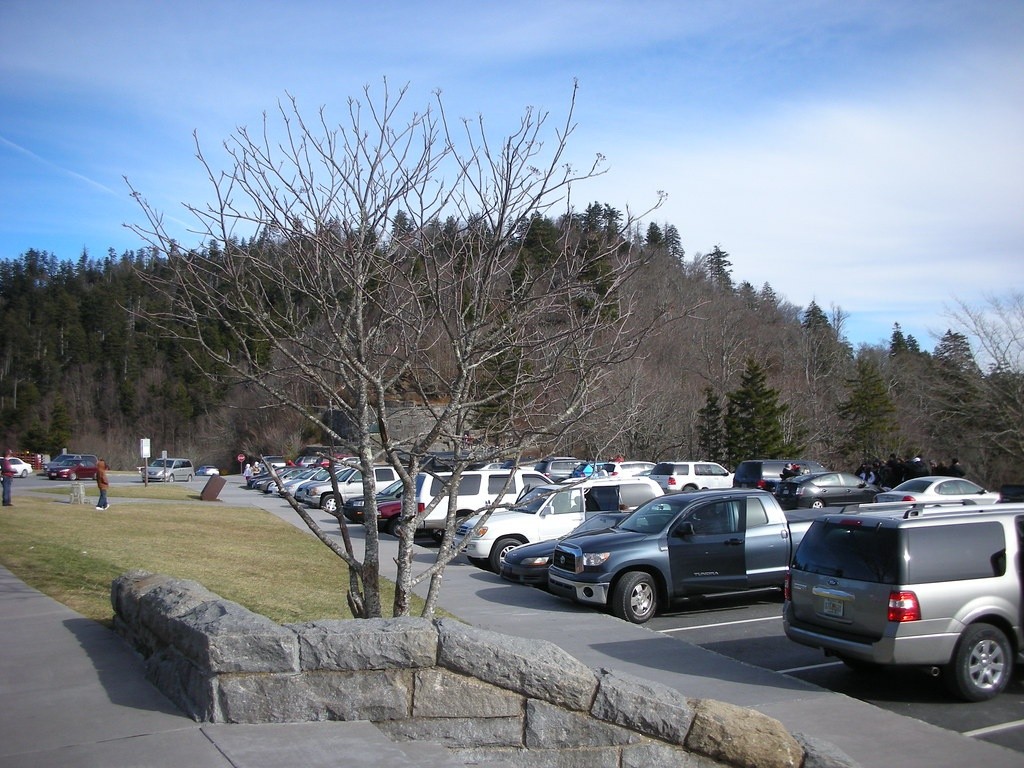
[999,483,1024,503]
[195,465,219,476]
[246,450,659,547]
[0,457,33,478]
[773,471,893,510]
[874,476,1001,507]
[499,507,678,586]
[47,458,98,481]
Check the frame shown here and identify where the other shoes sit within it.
[95,507,104,510]
[3,503,13,506]
[104,504,110,509]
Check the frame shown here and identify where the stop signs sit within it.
[237,454,245,463]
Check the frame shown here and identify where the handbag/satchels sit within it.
[246,468,253,476]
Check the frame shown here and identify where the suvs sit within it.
[782,497,1024,703]
[141,458,194,483]
[649,460,736,493]
[452,474,673,576]
[43,453,98,476]
[732,458,841,496]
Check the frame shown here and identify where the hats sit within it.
[255,461,259,465]
[245,464,250,468]
[5,448,13,454]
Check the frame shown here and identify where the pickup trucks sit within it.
[546,485,861,625]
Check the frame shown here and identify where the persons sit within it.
[783,463,800,480]
[95,461,110,510]
[855,453,966,491]
[243,461,261,481]
[286,459,303,467]
[0,453,17,506]
